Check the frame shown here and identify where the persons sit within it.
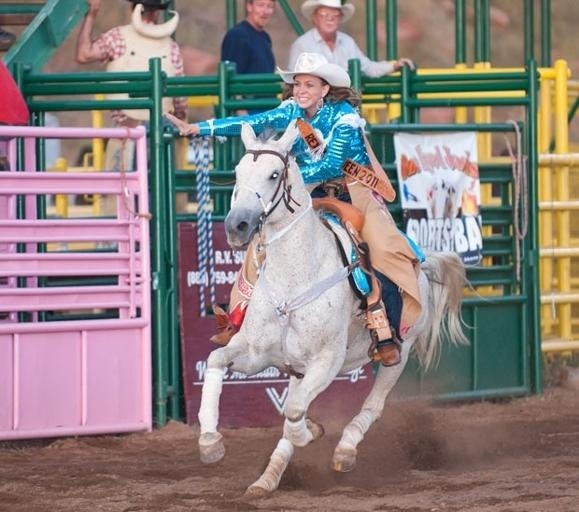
[75,0,189,131]
[288,1,415,78]
[1,60,29,141]
[220,0,277,117]
[163,52,402,366]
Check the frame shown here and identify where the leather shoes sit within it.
[210,326,235,346]
[378,345,401,366]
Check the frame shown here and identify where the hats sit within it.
[301,0,355,22]
[277,53,351,87]
[129,0,172,9]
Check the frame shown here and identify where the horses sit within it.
[196,117,493,503]
